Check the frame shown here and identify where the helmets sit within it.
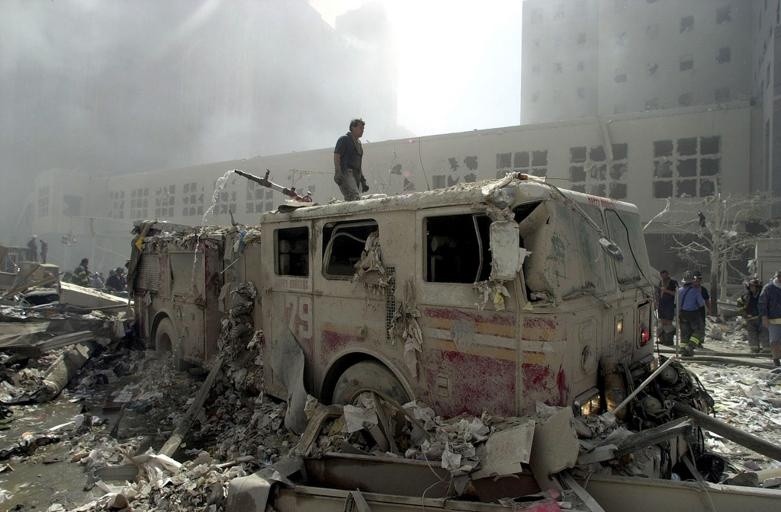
[682,271,697,283]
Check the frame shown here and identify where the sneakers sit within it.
[773,358,781,364]
[678,348,688,356]
[685,344,694,355]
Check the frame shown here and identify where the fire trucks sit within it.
[130,171,657,426]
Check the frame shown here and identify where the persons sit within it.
[655,270,676,345]
[671,270,706,357]
[757,270,781,366]
[27,234,38,261]
[693,270,713,350]
[333,117,370,201]
[39,239,49,264]
[63,257,128,296]
[736,278,772,355]
